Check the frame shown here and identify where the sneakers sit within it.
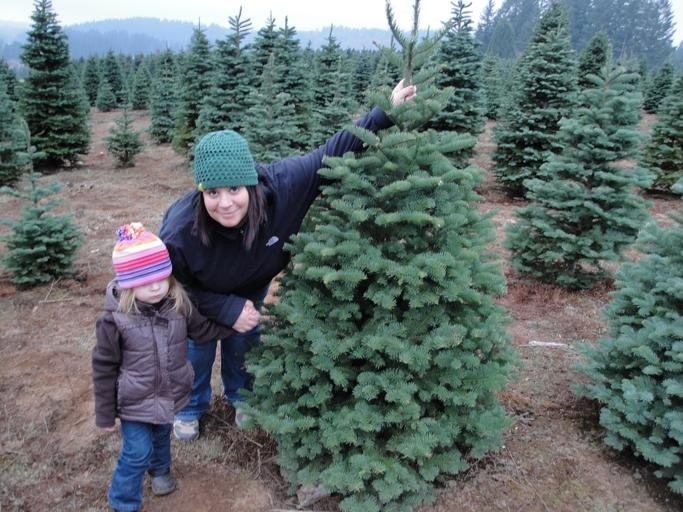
[150,470,175,496]
[235,406,252,427]
[172,418,200,440]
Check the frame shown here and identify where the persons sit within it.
[159,77,417,442]
[92,222,262,511]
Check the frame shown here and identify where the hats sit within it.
[193,130,258,191]
[112,222,172,288]
